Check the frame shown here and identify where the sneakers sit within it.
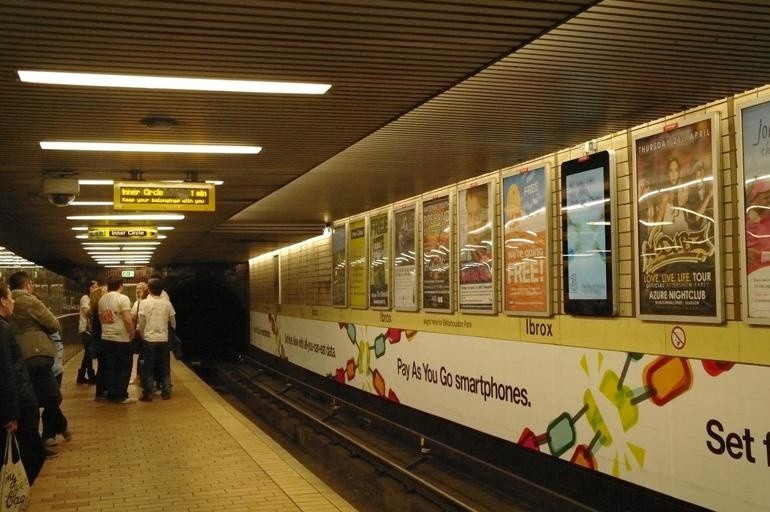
[41,446,60,459]
[139,395,152,402]
[118,397,138,404]
[160,391,172,401]
[45,436,58,447]
[95,391,110,400]
[61,426,71,441]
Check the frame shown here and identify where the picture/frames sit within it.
[626,110,728,324]
[368,208,391,311]
[500,161,553,318]
[330,218,348,309]
[560,148,622,319]
[420,190,454,314]
[347,215,368,310]
[736,95,770,323]
[392,200,419,312]
[456,178,497,314]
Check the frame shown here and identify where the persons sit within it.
[97,275,139,403]
[1,283,47,487]
[142,274,172,395]
[655,157,692,239]
[136,279,178,400]
[75,279,98,384]
[460,186,493,272]
[27,291,73,446]
[681,158,713,248]
[7,271,63,457]
[127,282,149,387]
[86,286,110,401]
[638,179,664,241]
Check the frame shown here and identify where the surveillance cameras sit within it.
[43,179,80,207]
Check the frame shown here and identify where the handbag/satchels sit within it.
[0,458,33,512]
[131,331,143,354]
[80,330,94,345]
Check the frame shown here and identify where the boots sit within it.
[74,368,90,384]
[87,370,97,386]
